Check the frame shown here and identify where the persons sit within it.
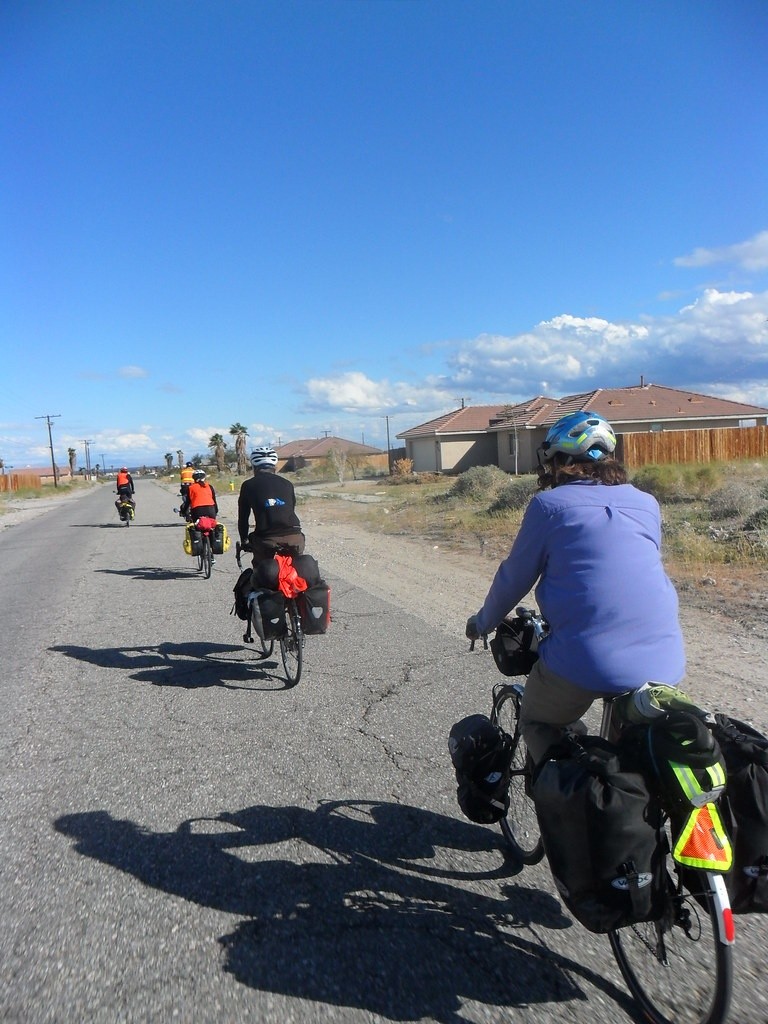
[116,467,134,499]
[465,411,690,766]
[238,447,306,648]
[183,470,219,564]
[180,462,196,502]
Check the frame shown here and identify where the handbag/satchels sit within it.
[183,515,231,556]
[115,499,135,520]
[232,554,330,641]
[533,688,768,934]
[449,714,511,824]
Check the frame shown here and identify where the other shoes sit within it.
[210,554,216,564]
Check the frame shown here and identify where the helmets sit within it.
[543,411,617,456]
[120,467,128,471]
[249,446,278,467]
[186,462,192,467]
[192,469,205,478]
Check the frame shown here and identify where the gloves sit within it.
[466,614,488,641]
[132,491,135,494]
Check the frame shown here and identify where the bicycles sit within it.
[469,606,738,1024]
[116,491,132,528]
[180,510,213,579]
[177,492,191,522]
[233,539,306,686]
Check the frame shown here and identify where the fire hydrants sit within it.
[229,480,235,492]
